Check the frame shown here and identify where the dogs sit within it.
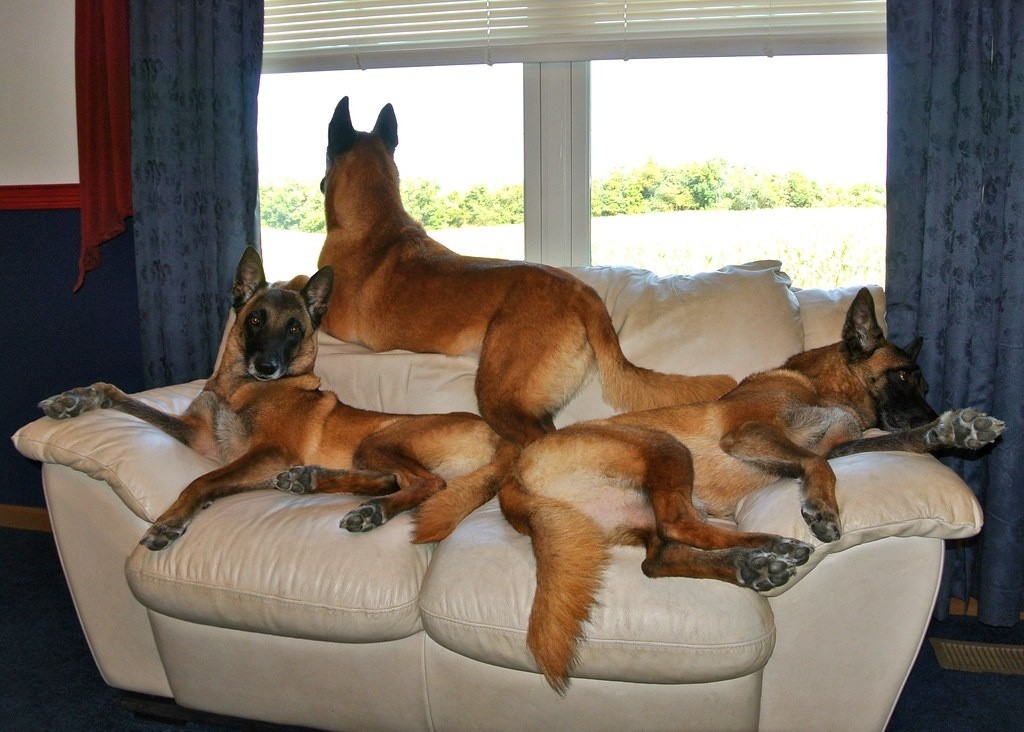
[495,287,1007,696]
[271,96,738,447]
[38,247,520,551]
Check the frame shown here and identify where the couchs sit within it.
[9,260,982,732]
